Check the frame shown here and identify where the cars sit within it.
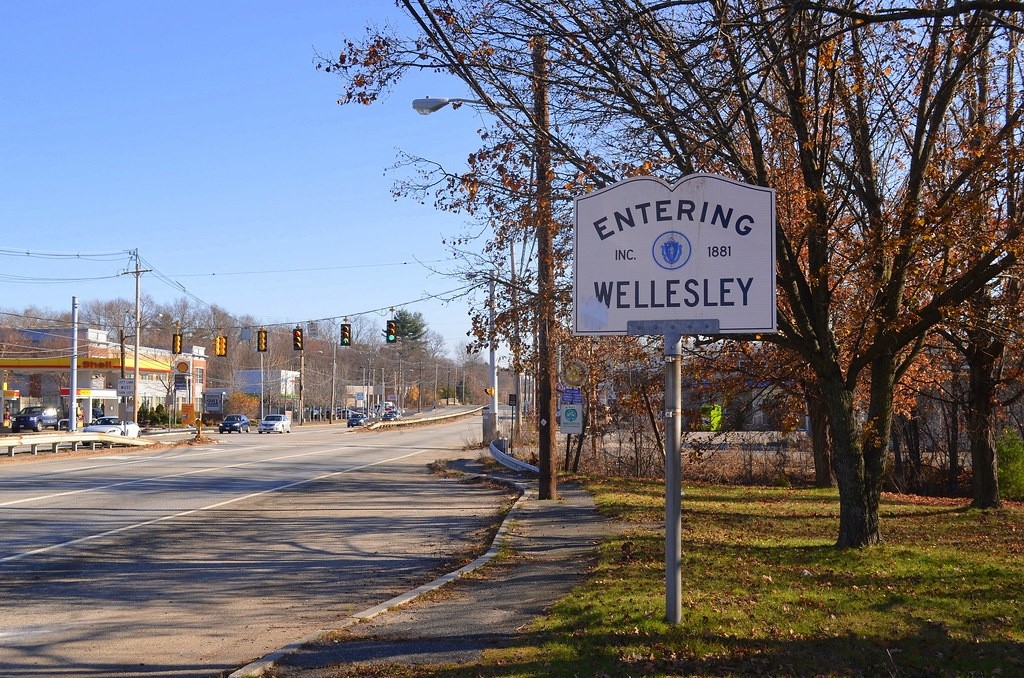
[219,414,251,434]
[83,415,142,438]
[306,394,399,419]
[91,408,105,419]
[258,413,291,434]
[382,412,398,421]
[347,413,367,428]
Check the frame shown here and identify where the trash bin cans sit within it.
[700,404,722,432]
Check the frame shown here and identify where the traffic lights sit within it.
[386,320,396,342]
[341,324,350,346]
[484,386,495,397]
[292,328,303,350]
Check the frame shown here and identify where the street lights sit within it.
[397,362,413,409]
[298,350,323,426]
[464,269,499,431]
[133,313,163,427]
[412,95,558,502]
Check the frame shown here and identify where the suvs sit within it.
[11,406,63,433]
